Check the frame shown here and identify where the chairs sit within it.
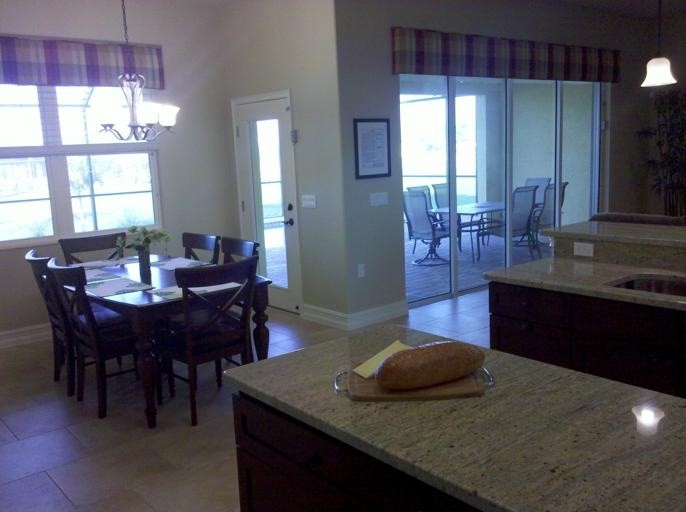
[52,230,127,265]
[511,176,552,248]
[431,180,469,253]
[401,190,452,267]
[178,228,220,268]
[154,253,258,427]
[532,182,570,240]
[220,235,260,271]
[407,184,445,252]
[481,184,536,262]
[23,246,124,398]
[51,262,177,421]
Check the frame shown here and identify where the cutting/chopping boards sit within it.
[335,360,495,402]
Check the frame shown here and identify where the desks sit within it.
[425,199,508,259]
[52,253,275,431]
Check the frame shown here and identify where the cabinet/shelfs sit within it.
[227,386,470,512]
[487,277,567,366]
[569,296,686,394]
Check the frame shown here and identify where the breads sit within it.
[378,342,486,390]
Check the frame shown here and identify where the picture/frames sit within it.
[352,116,392,180]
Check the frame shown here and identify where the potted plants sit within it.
[112,223,170,284]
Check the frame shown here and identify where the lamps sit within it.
[638,0,678,88]
[89,1,182,143]
